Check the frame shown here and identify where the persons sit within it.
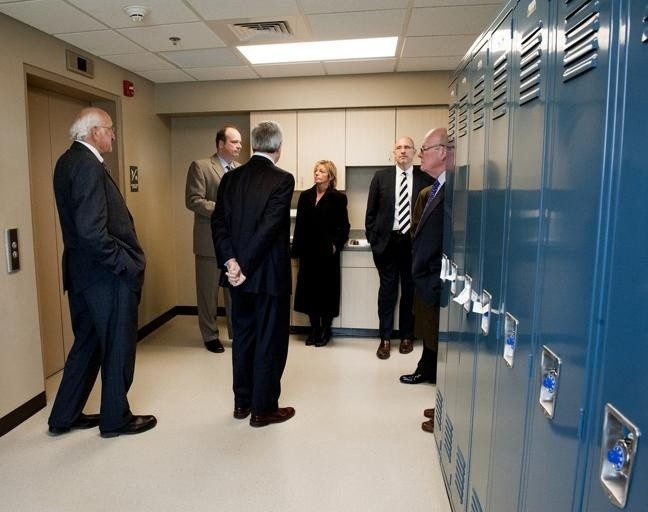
[49,107,158,439]
[211,122,296,428]
[420,126,455,434]
[286,160,350,348]
[398,185,439,385]
[364,136,418,359]
[186,124,246,353]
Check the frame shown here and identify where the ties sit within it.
[428,180,440,207]
[226,166,230,170]
[399,172,412,234]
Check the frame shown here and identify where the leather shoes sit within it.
[49,413,100,436]
[400,368,436,384]
[305,328,331,347]
[400,339,413,353]
[234,406,295,427]
[206,338,224,353]
[99,415,157,438]
[422,408,434,433]
[377,340,391,359]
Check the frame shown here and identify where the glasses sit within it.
[91,125,115,134]
[420,144,446,155]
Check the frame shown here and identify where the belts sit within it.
[392,231,411,235]
